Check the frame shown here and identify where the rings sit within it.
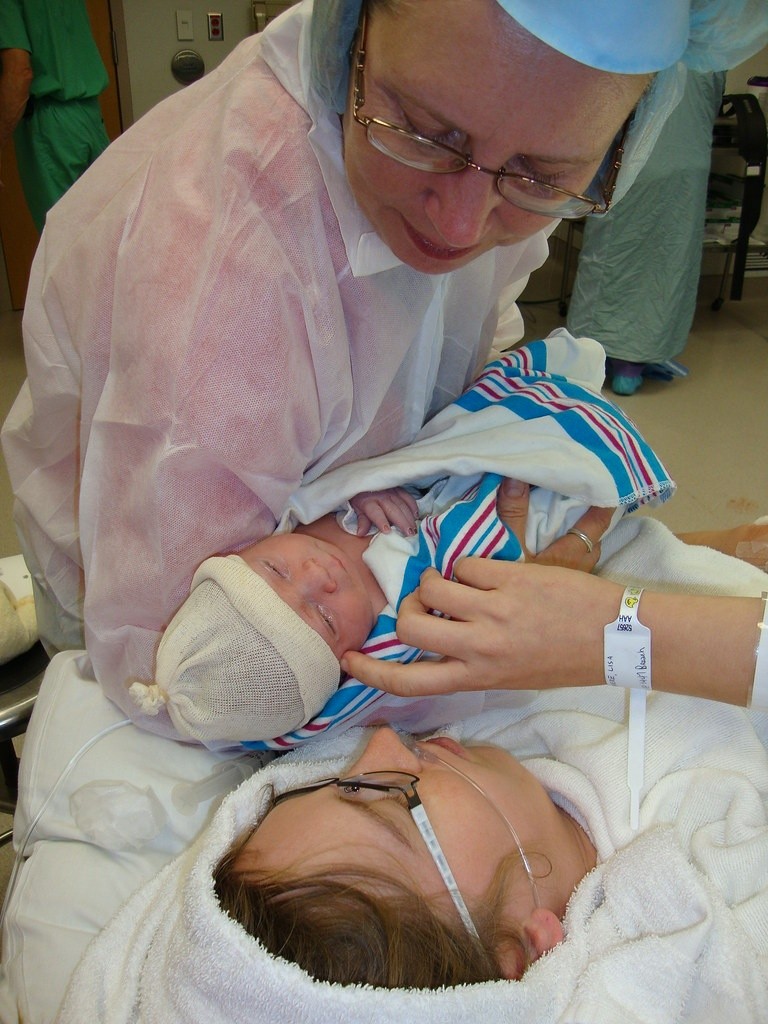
[568,527,596,551]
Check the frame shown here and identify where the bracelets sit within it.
[602,585,652,692]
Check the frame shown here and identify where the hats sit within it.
[129,554,341,741]
[497,0,691,75]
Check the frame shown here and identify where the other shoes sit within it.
[613,371,643,395]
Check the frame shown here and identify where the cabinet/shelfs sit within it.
[557,95,768,318]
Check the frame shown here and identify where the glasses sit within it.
[272,770,487,961]
[351,14,628,221]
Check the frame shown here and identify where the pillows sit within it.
[0,650,261,1024]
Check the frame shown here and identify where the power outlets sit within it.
[207,12,224,41]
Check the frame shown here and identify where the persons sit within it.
[3,0,691,739]
[0,0,112,233]
[565,1,726,397]
[168,551,768,1023]
[125,328,673,742]
